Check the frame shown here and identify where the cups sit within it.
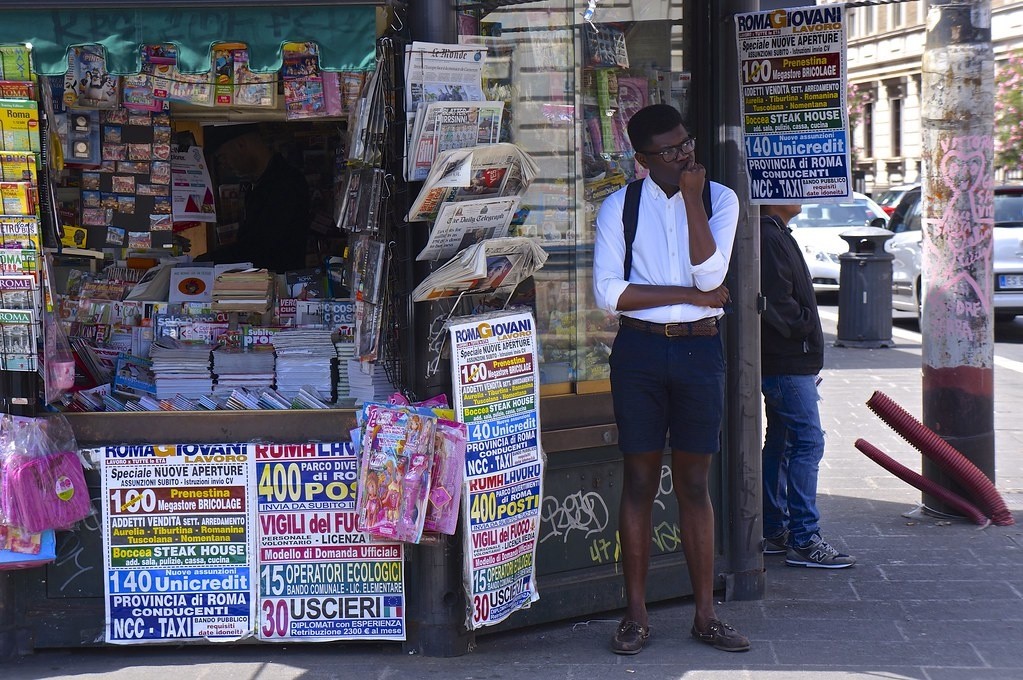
[47,358,75,390]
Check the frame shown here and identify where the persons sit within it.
[591,103,753,654]
[450,258,511,295]
[761,204,857,568]
[192,124,311,274]
[359,414,448,546]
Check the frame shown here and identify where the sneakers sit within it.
[761,538,787,553]
[786,537,856,568]
[611,618,650,654]
[691,619,751,651]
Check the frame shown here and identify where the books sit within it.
[50,239,388,417]
[0,44,44,372]
[44,40,386,250]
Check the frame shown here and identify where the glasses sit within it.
[639,134,697,163]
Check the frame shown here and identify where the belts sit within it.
[621,316,718,337]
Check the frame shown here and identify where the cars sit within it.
[781,192,891,307]
[870,179,1023,332]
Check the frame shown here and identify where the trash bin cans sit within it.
[831,232,896,350]
